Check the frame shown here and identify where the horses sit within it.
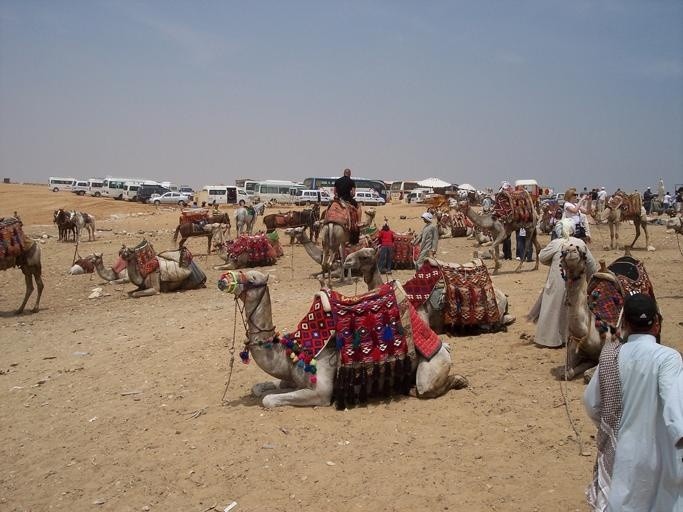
[173,207,231,255]
[235,202,267,239]
[263,207,295,244]
[284,201,321,244]
[53,209,96,242]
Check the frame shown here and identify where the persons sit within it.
[580,186,683,216]
[582,215,592,243]
[191,202,198,208]
[579,289,682,511]
[501,237,512,259]
[410,213,439,276]
[334,169,363,226]
[548,209,562,242]
[562,188,593,243]
[200,201,206,208]
[515,229,533,262]
[376,222,394,275]
[481,194,491,211]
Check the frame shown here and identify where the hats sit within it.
[420,212,435,224]
[625,293,658,325]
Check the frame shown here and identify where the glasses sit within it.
[572,193,579,197]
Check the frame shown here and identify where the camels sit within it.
[284,196,421,288]
[217,270,469,408]
[559,243,661,384]
[222,224,284,257]
[0,236,44,313]
[344,244,515,336]
[84,247,207,297]
[667,217,683,235]
[427,184,664,272]
[202,222,278,270]
